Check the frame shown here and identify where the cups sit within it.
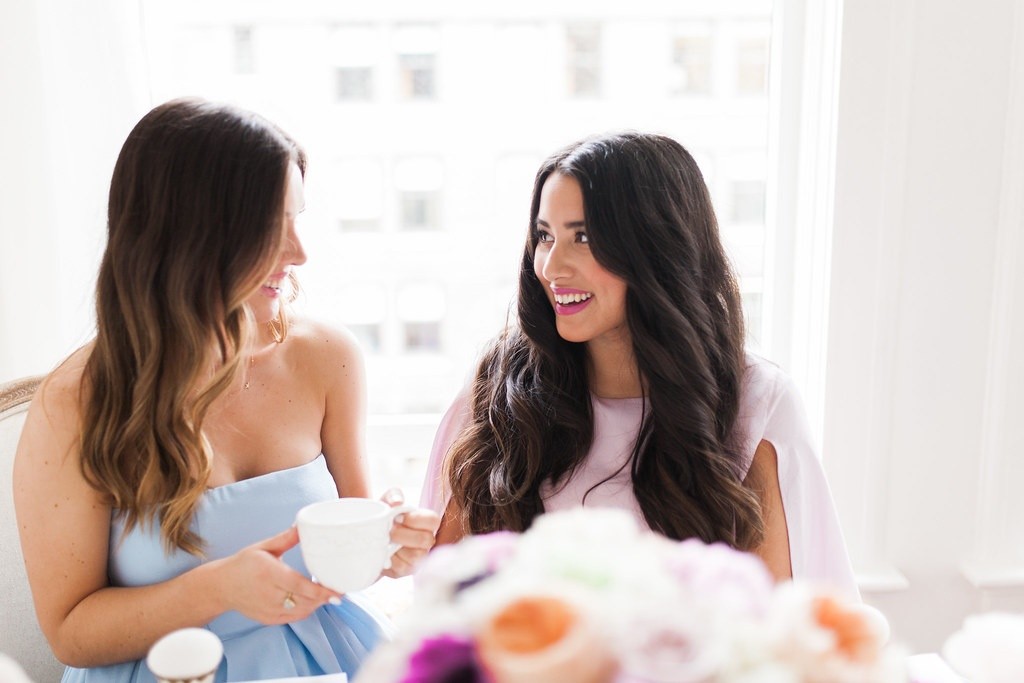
[297,497,412,593]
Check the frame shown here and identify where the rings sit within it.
[283,592,295,611]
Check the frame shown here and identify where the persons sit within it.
[416,133,863,607]
[12,98,438,683]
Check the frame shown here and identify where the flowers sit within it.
[347,505,910,683]
